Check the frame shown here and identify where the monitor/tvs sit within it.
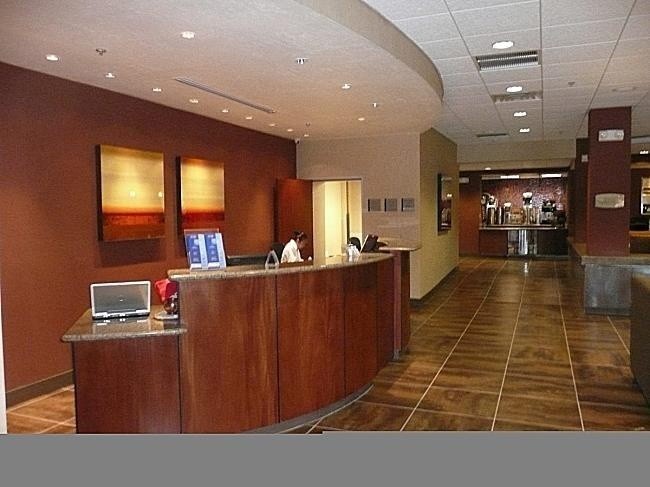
[360,234,378,253]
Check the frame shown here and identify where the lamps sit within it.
[598,129,624,142]
[595,193,625,209]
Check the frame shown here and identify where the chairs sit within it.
[630,273,650,405]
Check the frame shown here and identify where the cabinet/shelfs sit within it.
[537,229,569,256]
[479,226,508,258]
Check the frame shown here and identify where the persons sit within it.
[281,230,308,263]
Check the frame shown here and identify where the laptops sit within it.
[91,317,151,334]
[90,281,151,318]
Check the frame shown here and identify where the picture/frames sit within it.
[438,173,451,231]
